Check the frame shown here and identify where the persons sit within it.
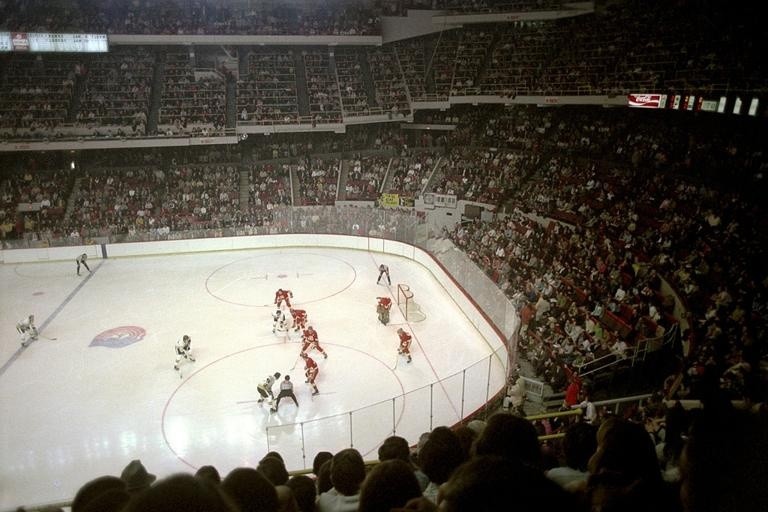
[300,352,319,397]
[270,374,300,413]
[300,326,329,360]
[74,252,92,277]
[396,327,413,364]
[0,1,767,249]
[273,287,295,310]
[467,249,767,512]
[173,333,196,371]
[63,422,466,512]
[291,306,309,333]
[17,314,40,348]
[271,308,292,345]
[256,371,281,406]
[375,263,392,286]
[376,296,393,324]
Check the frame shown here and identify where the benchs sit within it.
[0,2,768,512]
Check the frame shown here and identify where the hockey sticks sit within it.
[39,335,57,341]
[289,355,298,371]
[390,353,399,371]
[264,297,292,307]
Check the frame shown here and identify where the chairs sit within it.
[506,209,679,382]
